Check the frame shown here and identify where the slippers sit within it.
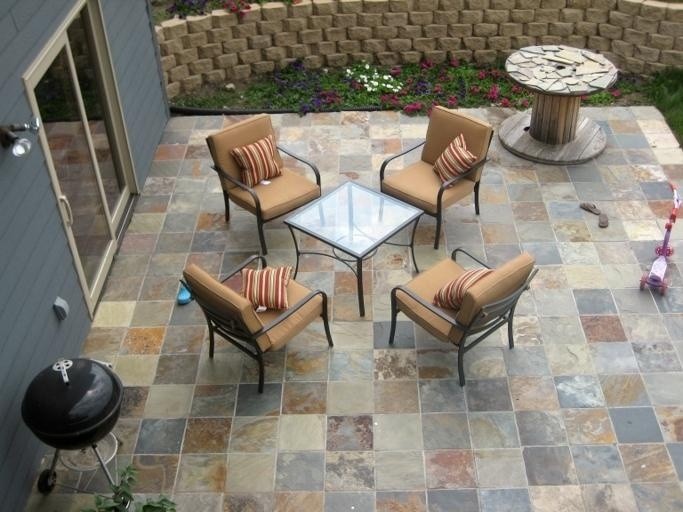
[579,202,601,215]
[599,213,609,228]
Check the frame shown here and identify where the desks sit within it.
[281,179,424,317]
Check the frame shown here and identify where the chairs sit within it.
[378,104,495,251]
[389,247,538,385]
[203,113,327,255]
[180,250,333,392]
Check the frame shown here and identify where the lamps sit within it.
[0,117,48,162]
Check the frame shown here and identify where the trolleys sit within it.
[20,358,137,512]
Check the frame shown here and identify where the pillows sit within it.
[432,264,493,314]
[238,265,290,309]
[428,133,475,188]
[229,136,282,187]
